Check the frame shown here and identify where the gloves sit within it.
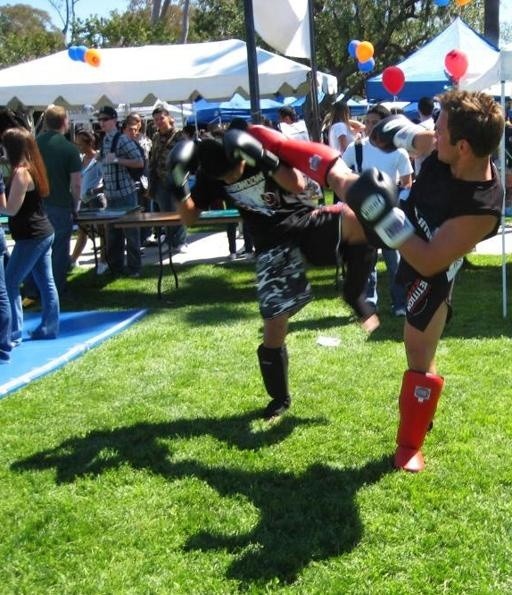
[163,138,203,204]
[223,127,280,175]
[343,167,418,251]
[368,111,426,155]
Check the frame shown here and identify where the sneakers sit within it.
[159,243,168,254]
[70,260,80,268]
[228,253,237,258]
[125,270,143,278]
[96,261,110,276]
[390,305,407,318]
[237,248,247,256]
[170,245,189,253]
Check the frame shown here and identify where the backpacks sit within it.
[352,137,368,175]
[98,130,145,186]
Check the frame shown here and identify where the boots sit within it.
[246,124,344,189]
[256,342,292,419]
[393,368,445,473]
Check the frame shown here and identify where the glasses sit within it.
[96,116,115,122]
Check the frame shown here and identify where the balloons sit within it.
[356,41,374,63]
[84,49,101,68]
[455,0,471,7]
[432,0,450,8]
[75,45,86,63]
[444,47,469,89]
[381,65,405,104]
[355,56,373,72]
[67,45,79,61]
[349,38,359,59]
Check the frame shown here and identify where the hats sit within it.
[93,106,118,118]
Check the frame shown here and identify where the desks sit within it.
[112,208,348,300]
[73,211,128,278]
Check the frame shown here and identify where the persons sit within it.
[245,88,510,471]
[69,129,114,276]
[166,131,388,429]
[88,105,150,278]
[328,99,360,154]
[335,98,420,334]
[128,111,152,155]
[0,123,59,345]
[32,104,82,296]
[116,118,146,175]
[0,174,15,364]
[149,105,198,257]
[406,91,440,172]
[221,115,256,263]
[277,104,310,155]
